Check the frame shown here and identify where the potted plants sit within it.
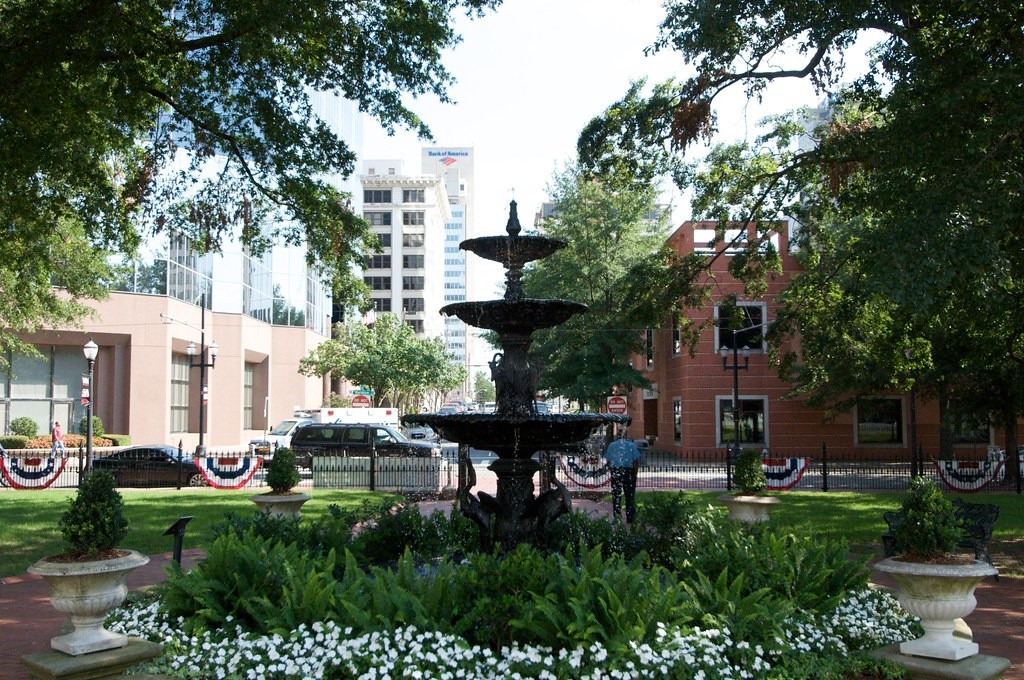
[872,476,997,662]
[248,446,313,519]
[716,449,781,526]
[27,467,150,656]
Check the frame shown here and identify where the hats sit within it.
[55,422,58,424]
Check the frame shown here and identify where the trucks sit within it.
[249,408,399,465]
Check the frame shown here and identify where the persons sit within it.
[51,421,66,459]
[604,427,641,524]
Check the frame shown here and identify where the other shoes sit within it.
[62,457,68,461]
[626,517,635,523]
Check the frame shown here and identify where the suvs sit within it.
[289,421,441,469]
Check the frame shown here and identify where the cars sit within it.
[437,400,551,417]
[989,445,1024,483]
[404,422,436,440]
[82,445,211,487]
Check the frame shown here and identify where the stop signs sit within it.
[351,396,371,408]
[607,396,627,415]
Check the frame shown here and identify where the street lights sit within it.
[82,338,100,485]
[720,345,752,487]
[186,290,219,458]
